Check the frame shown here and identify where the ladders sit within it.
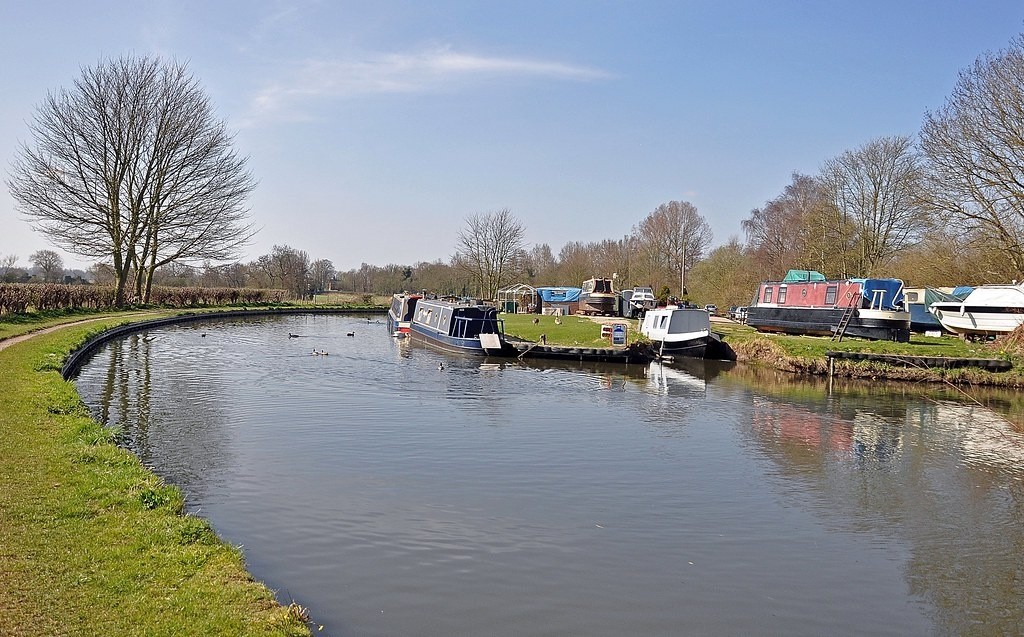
[831,292,861,343]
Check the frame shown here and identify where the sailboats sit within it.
[641,242,712,359]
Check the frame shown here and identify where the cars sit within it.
[703,304,718,317]
[728,305,748,321]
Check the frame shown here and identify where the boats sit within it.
[387,292,425,339]
[409,289,504,357]
[743,272,911,345]
[629,286,657,311]
[931,283,1023,337]
[577,275,620,304]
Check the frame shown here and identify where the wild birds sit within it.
[554,312,562,325]
[366,316,379,325]
[288,332,299,337]
[312,348,328,355]
[347,331,355,336]
[437,362,444,370]
[201,332,206,338]
[531,318,539,326]
